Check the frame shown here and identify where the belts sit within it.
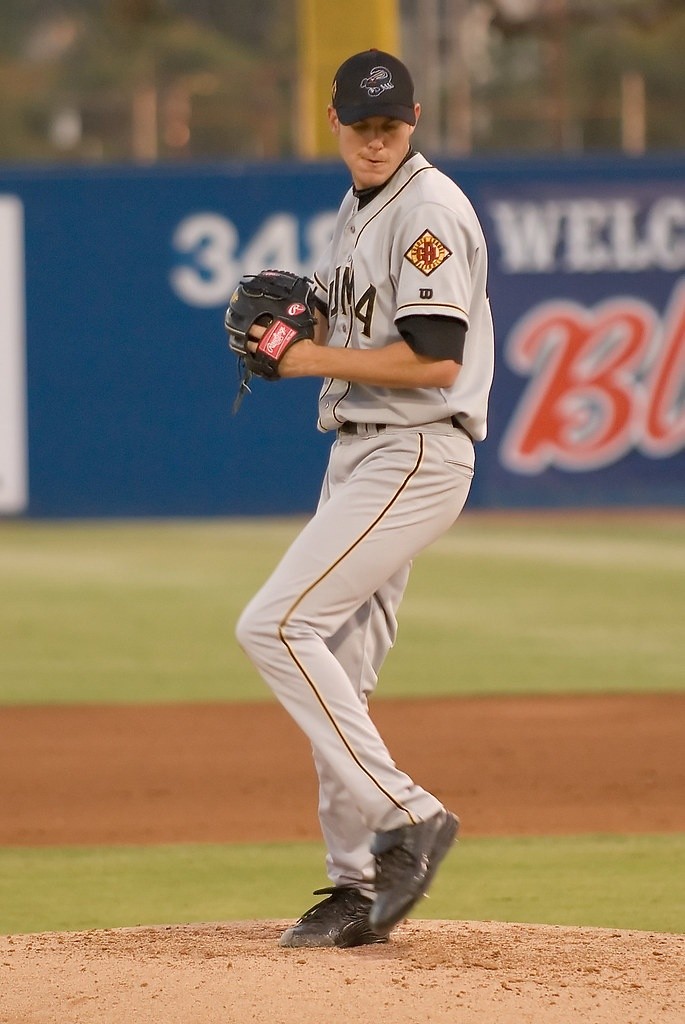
[338,415,464,433]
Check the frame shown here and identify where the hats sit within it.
[331,48,416,126]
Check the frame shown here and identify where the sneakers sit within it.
[278,886,390,947]
[368,807,461,936]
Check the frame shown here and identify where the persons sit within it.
[223,47,495,948]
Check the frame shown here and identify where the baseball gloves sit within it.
[225,268,317,381]
